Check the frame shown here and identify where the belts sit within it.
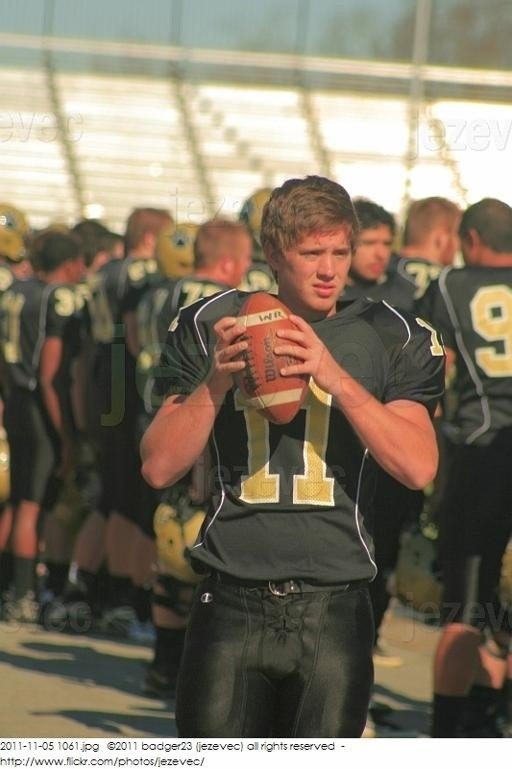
[208,567,370,597]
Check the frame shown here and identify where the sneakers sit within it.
[139,662,174,698]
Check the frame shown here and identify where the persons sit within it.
[0,176,512,738]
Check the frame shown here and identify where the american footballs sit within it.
[239,293,309,423]
